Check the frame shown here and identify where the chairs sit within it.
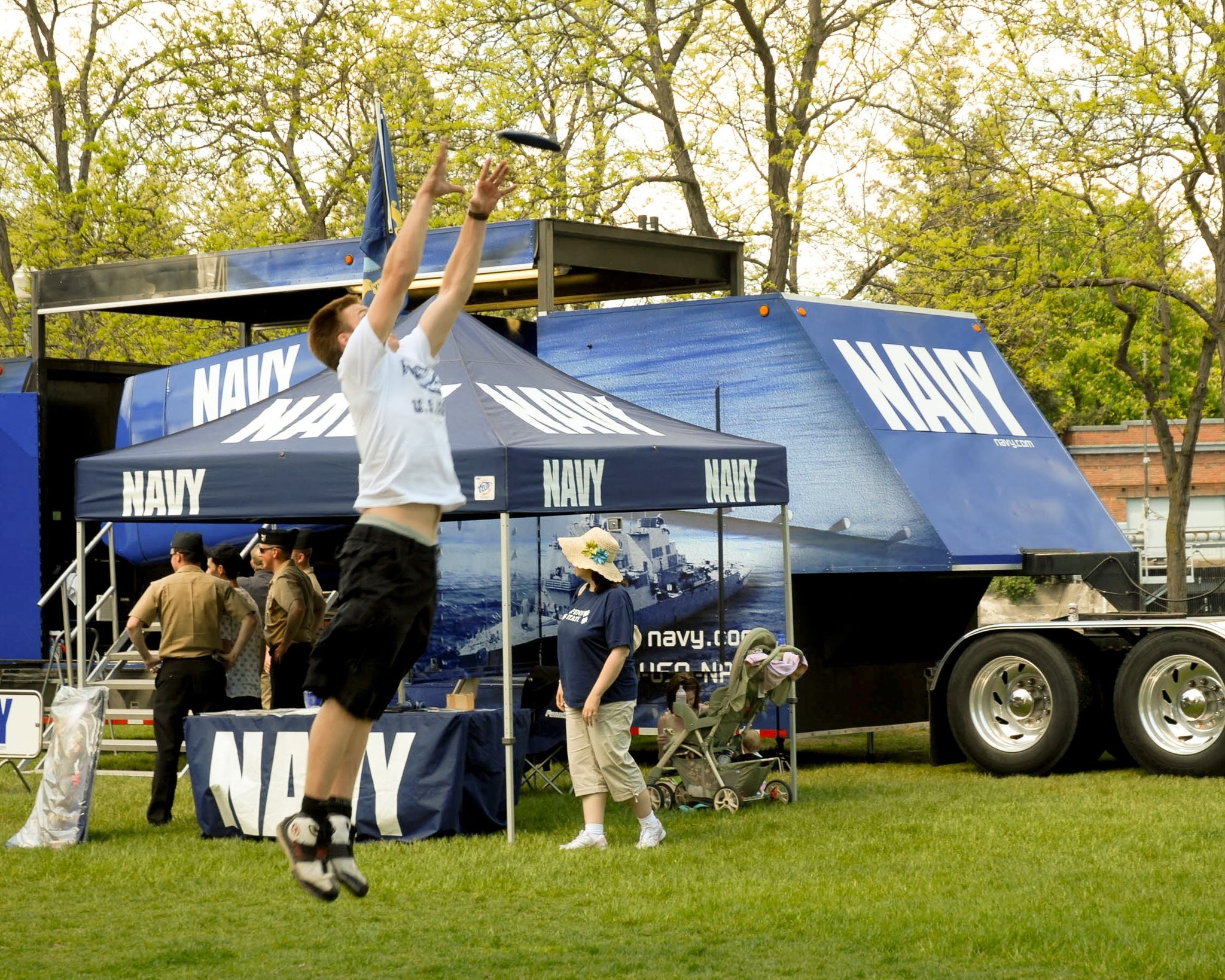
[521,665,574,795]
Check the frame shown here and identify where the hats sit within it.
[294,530,313,549]
[557,527,624,582]
[257,528,299,549]
[171,532,203,555]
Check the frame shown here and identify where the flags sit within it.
[359,104,407,315]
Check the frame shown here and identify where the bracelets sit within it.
[467,206,490,221]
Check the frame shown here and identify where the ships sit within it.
[459,513,754,674]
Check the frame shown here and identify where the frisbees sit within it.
[498,129,562,153]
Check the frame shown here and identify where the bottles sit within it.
[718,748,735,764]
[675,686,686,702]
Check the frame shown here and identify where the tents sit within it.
[73,294,798,848]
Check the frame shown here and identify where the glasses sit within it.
[259,547,284,554]
[170,551,190,560]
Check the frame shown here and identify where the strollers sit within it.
[645,626,807,815]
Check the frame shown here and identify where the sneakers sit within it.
[560,829,609,849]
[277,813,369,901]
[635,819,666,849]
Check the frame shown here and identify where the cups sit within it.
[303,690,322,707]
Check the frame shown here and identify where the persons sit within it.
[554,526,666,850]
[656,673,761,782]
[279,133,518,901]
[126,528,327,824]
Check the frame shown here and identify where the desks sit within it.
[184,708,530,843]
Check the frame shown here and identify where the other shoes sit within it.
[201,832,222,839]
